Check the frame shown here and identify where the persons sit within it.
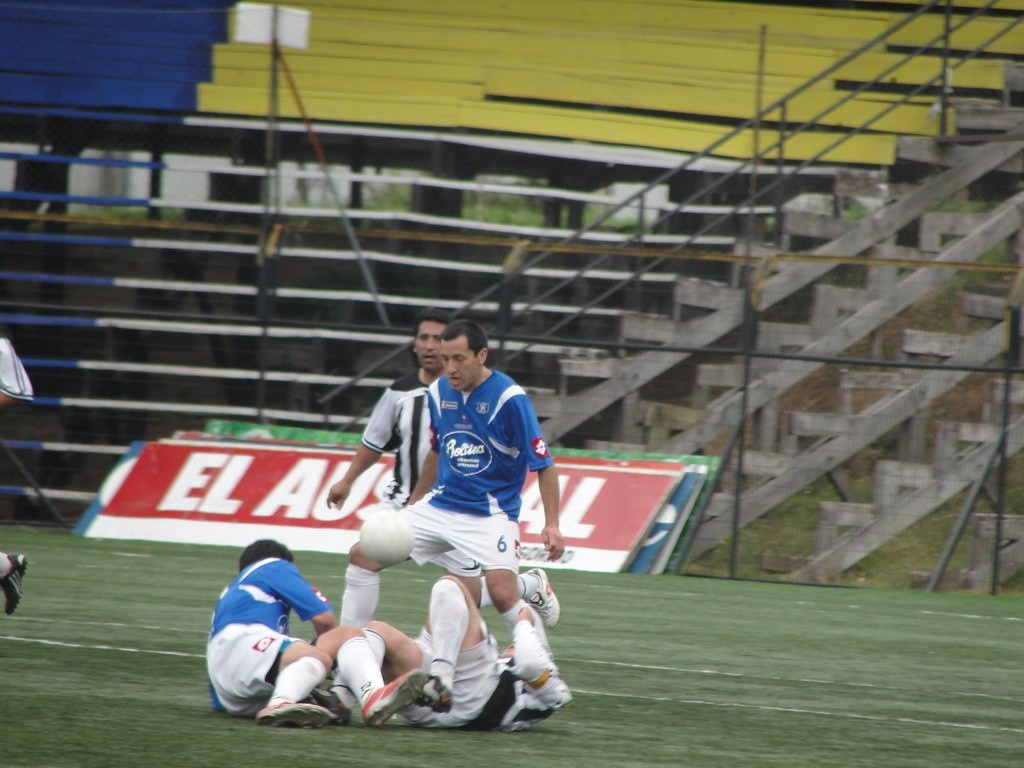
[206,310,574,731]
[0,334,34,615]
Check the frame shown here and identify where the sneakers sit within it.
[414,673,452,713]
[524,568,560,629]
[362,669,426,727]
[306,688,351,726]
[255,701,330,728]
[1,553,27,614]
